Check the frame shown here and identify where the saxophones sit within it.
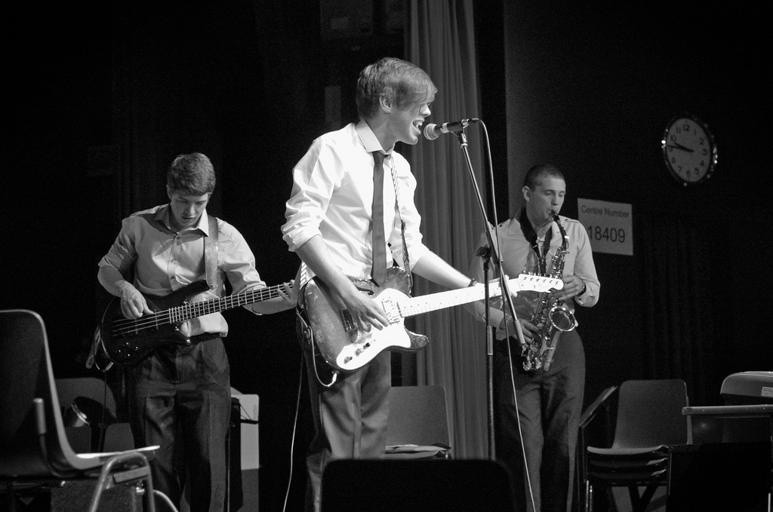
[522,210,578,373]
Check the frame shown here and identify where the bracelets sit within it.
[468,277,478,293]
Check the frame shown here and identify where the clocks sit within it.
[656,111,720,196]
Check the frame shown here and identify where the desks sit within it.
[682,400,768,508]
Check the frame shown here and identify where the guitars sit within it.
[298,271,564,373]
[100,279,293,365]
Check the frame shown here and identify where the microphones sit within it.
[422,113,469,142]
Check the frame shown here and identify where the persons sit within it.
[280,57,518,511]
[97,152,299,511]
[462,166,602,510]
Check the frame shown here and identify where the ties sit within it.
[371,151,387,286]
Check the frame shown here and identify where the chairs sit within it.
[565,376,693,512]
[383,383,450,458]
